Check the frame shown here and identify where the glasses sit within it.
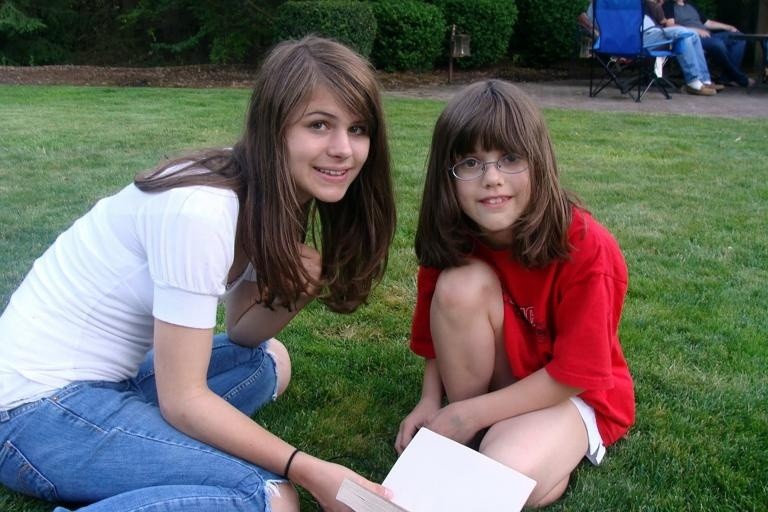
[452,151,529,181]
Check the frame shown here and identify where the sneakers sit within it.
[686,76,756,96]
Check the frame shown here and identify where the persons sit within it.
[661,0,756,91]
[0,36,399,512]
[392,79,636,509]
[583,0,727,97]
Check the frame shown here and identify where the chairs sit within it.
[579,1,682,104]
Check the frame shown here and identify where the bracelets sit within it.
[280,445,305,479]
